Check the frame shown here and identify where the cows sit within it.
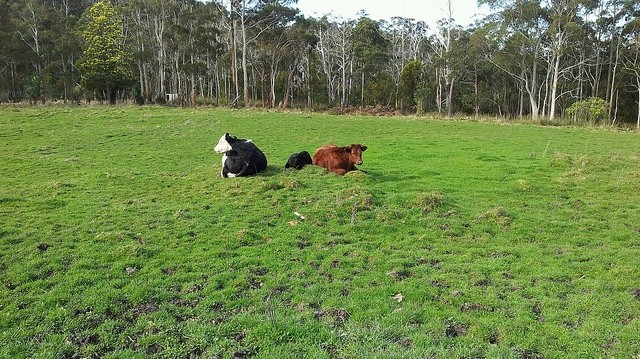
[214,133,267,179]
[311,143,368,176]
[284,150,313,170]
